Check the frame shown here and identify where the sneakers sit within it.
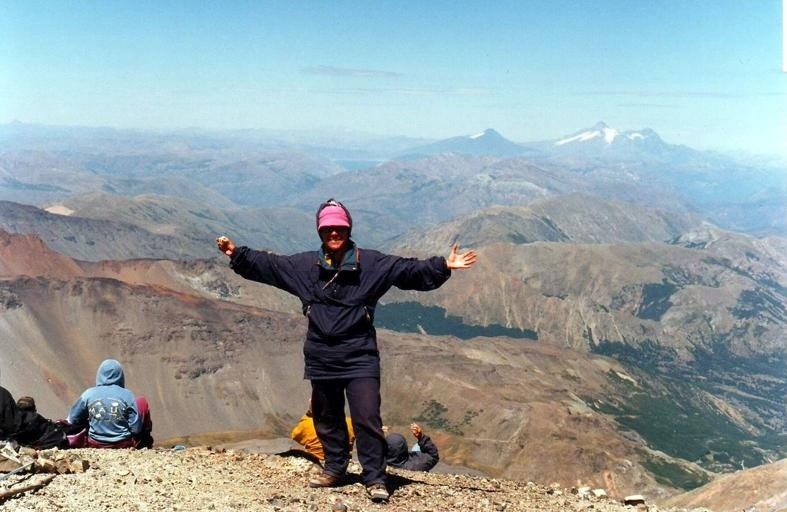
[364,481,389,501]
[309,473,341,488]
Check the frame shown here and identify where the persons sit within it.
[16,396,67,451]
[68,358,153,449]
[216,198,478,502]
[289,396,356,467]
[380,423,440,472]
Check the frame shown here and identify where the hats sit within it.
[18,396,35,405]
[317,205,350,231]
